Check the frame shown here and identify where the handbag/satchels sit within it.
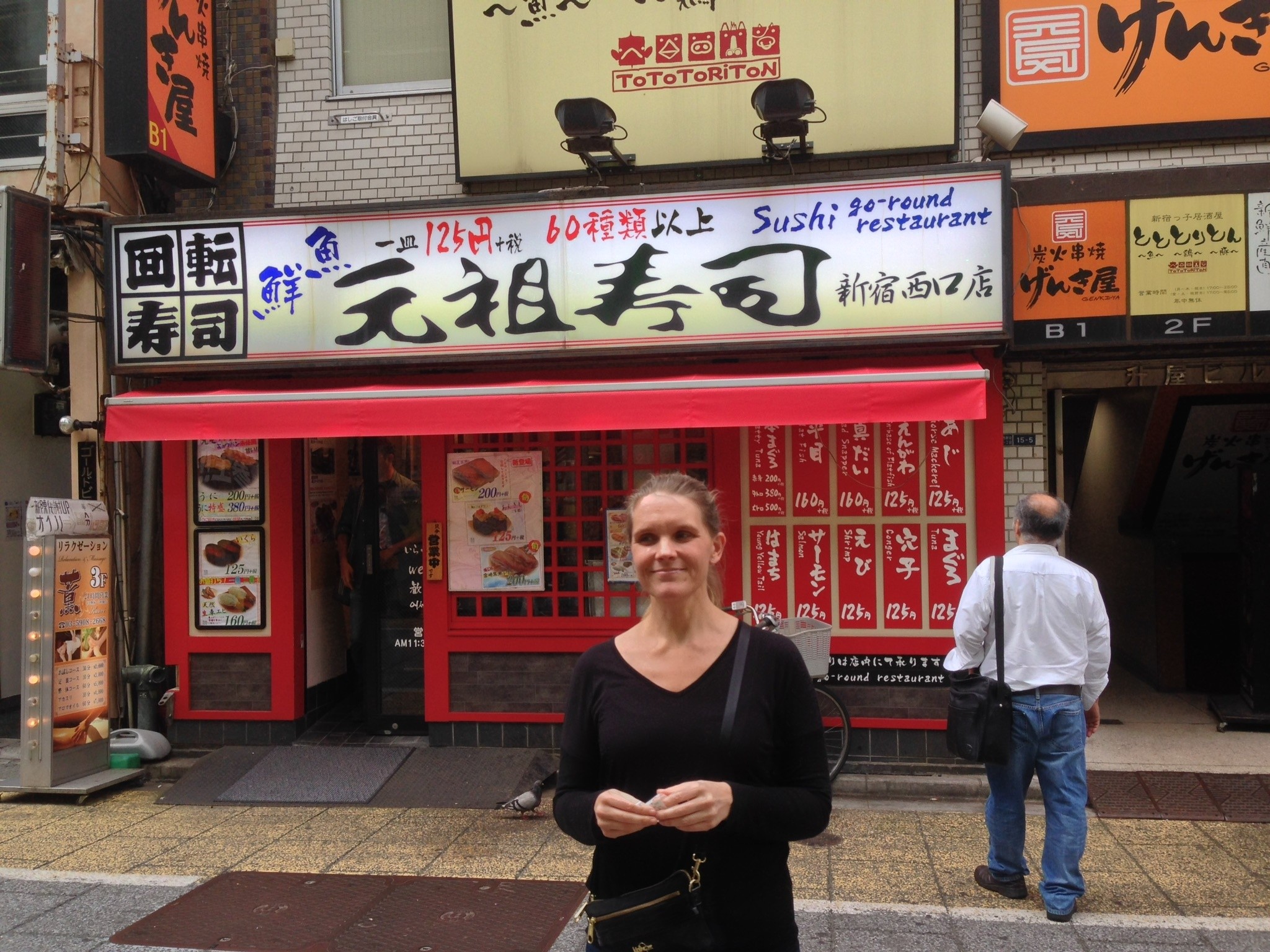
[946,669,1013,766]
[584,869,718,952]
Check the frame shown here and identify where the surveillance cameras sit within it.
[975,97,1029,159]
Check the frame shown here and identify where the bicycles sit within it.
[725,602,849,784]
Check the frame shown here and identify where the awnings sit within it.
[103,352,990,442]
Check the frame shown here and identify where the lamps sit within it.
[751,80,827,160]
[554,98,636,174]
[970,99,1029,162]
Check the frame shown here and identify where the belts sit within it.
[1011,684,1082,698]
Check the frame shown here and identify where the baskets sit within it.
[773,617,832,679]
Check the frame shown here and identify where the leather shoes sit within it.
[1047,898,1078,922]
[974,865,1027,899]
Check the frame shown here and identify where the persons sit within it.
[333,439,422,721]
[942,493,1111,922]
[550,474,832,952]
